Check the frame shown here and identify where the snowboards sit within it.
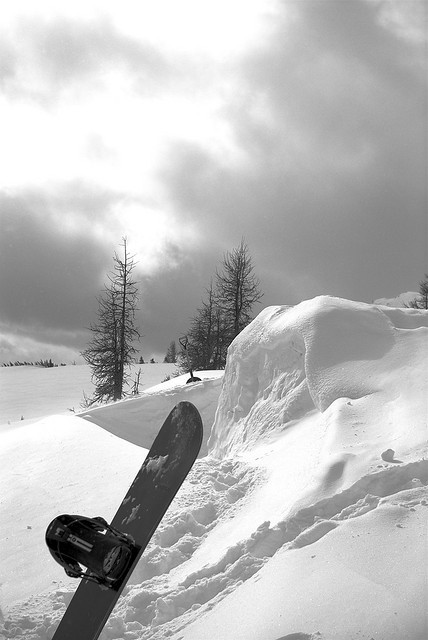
[51,401,203,640]
